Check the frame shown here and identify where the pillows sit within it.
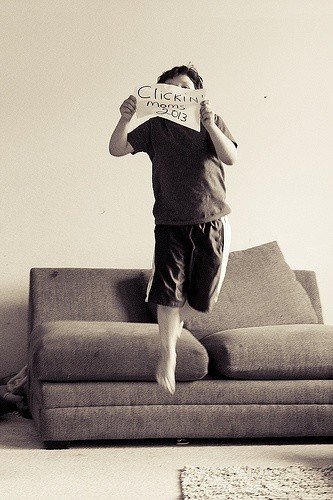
[200,324,333,380]
[178,240,320,341]
[30,320,208,382]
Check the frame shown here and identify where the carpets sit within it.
[181,466,333,500]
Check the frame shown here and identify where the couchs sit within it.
[29,269,333,450]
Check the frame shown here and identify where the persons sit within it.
[109,64,239,395]
